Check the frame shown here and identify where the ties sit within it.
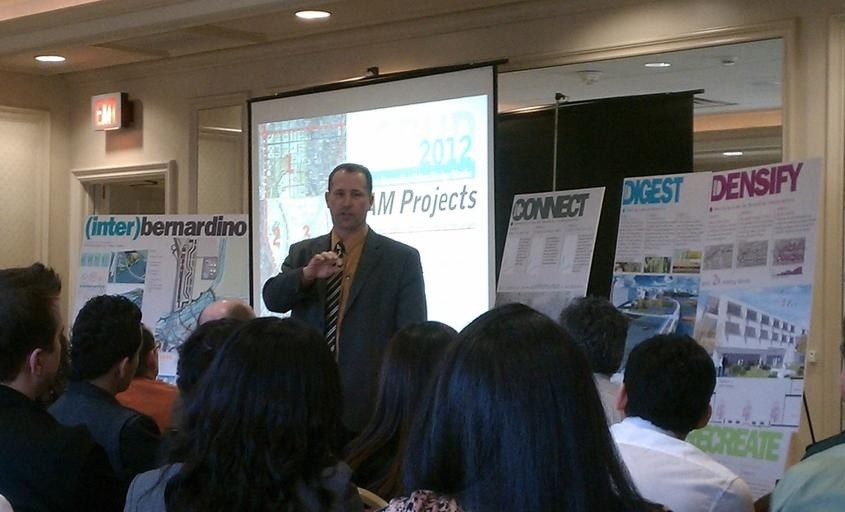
[325,241,345,359]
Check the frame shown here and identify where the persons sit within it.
[114,326,178,437]
[122,316,364,512]
[557,293,629,426]
[368,302,674,512]
[175,319,246,404]
[47,292,164,488]
[609,332,756,511]
[262,163,427,444]
[802,340,845,460]
[198,298,257,326]
[346,320,459,504]
[0,262,123,512]
[721,353,729,376]
